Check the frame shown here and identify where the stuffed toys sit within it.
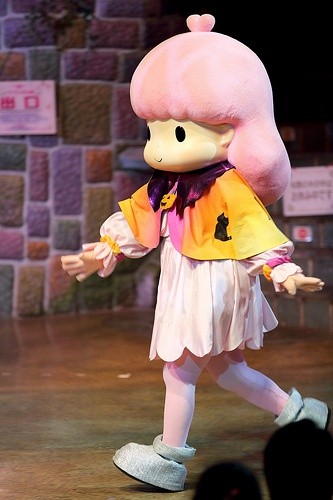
[56,13,329,492]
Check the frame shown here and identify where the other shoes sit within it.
[269,385,332,433]
[113,434,194,491]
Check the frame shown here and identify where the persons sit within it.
[187,455,264,499]
[260,418,333,500]
